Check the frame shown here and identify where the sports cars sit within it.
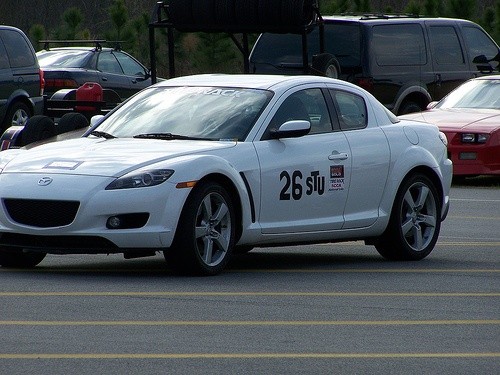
[0,73,454,277]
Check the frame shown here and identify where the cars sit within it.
[35,38,169,103]
[396,74,500,177]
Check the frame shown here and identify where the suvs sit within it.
[247,10,500,116]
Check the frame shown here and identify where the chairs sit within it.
[271,97,311,129]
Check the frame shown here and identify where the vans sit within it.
[0,25,46,129]
